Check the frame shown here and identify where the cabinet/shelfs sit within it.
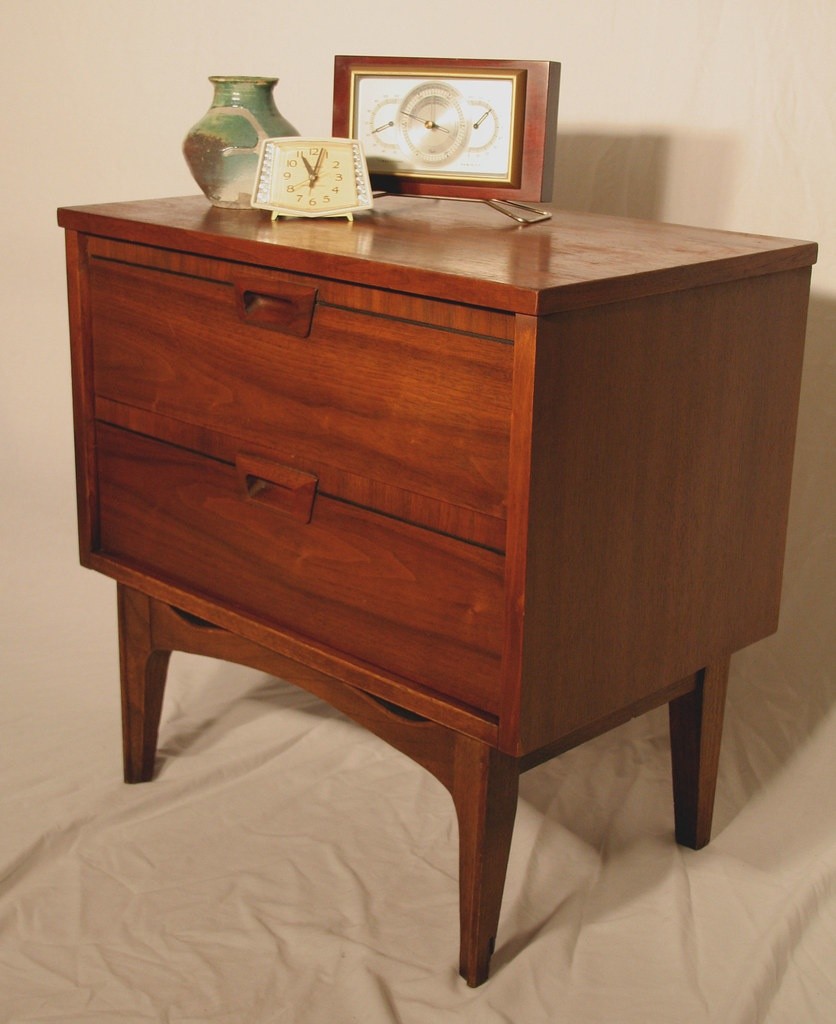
[55,197,818,988]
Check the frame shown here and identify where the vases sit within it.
[184,76,303,209]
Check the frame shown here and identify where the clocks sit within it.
[333,57,563,227]
[251,138,374,223]
